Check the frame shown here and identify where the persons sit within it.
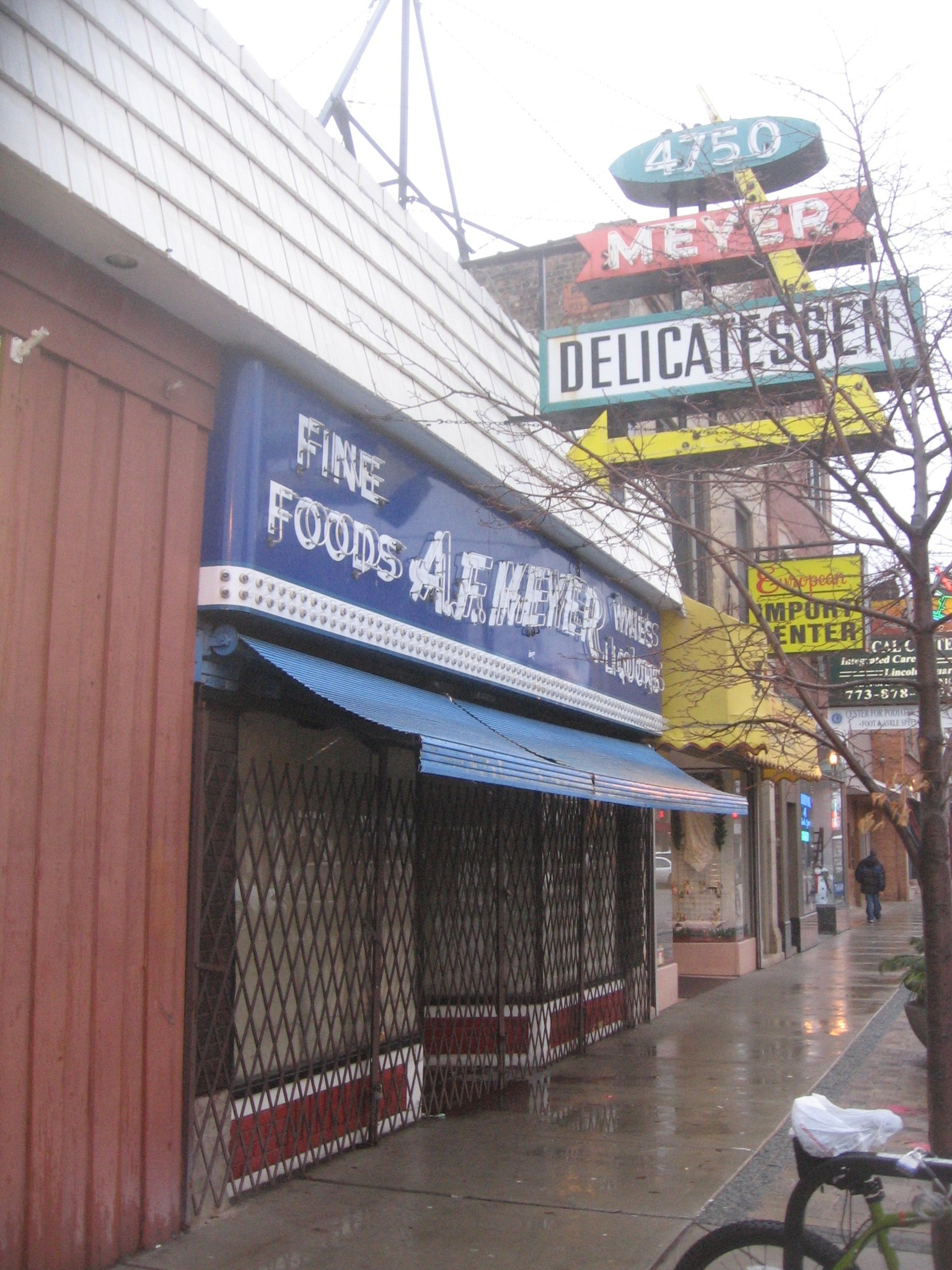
[855,849,888,923]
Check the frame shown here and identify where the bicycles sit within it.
[666,1094,949,1269]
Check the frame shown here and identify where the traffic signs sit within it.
[534,115,943,497]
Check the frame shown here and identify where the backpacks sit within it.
[861,873,879,894]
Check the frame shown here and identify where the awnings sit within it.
[229,623,751,826]
[659,589,825,787]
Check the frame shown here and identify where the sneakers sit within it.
[868,919,874,923]
